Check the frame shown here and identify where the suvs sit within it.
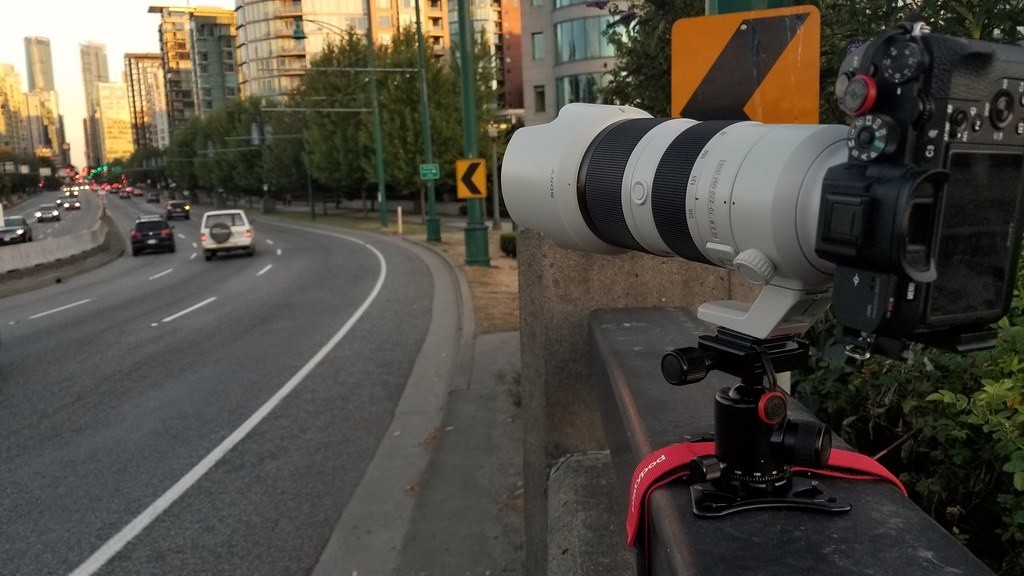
[200,209,255,262]
[131,215,175,256]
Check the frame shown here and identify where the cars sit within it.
[38,203,59,222]
[0,217,33,243]
[55,184,160,211]
[166,201,190,220]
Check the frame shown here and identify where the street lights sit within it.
[487,121,502,231]
[291,16,387,228]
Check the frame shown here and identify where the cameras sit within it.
[501,25,1024,351]
